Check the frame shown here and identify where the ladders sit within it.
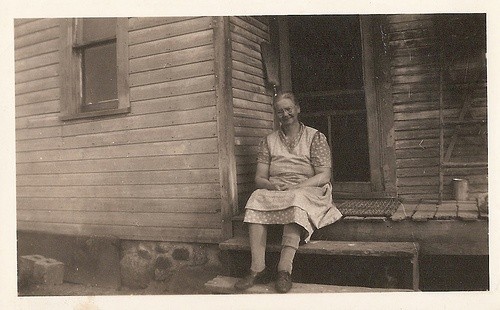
[435,13,488,204]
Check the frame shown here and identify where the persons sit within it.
[233,91,333,294]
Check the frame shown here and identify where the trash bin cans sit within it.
[451,176,470,202]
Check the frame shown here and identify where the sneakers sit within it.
[234,269,272,289]
[276,271,292,292]
